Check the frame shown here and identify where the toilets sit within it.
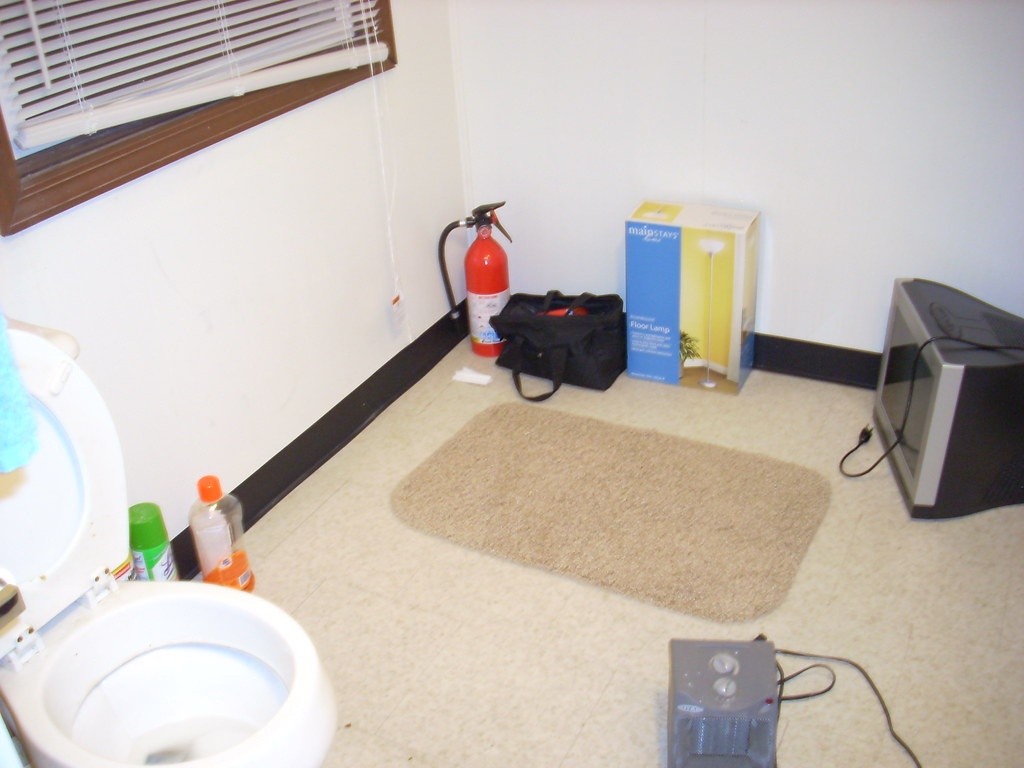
[0,325,336,768]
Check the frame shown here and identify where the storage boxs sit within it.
[624,201,760,395]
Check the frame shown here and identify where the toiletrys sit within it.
[187,474,255,595]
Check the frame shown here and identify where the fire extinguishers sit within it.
[438,201,514,358]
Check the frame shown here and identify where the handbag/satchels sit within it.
[489,289,627,402]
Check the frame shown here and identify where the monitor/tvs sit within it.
[872,276,1024,520]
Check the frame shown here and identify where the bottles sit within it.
[188,476,255,595]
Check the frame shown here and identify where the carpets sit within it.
[390,401,832,624]
